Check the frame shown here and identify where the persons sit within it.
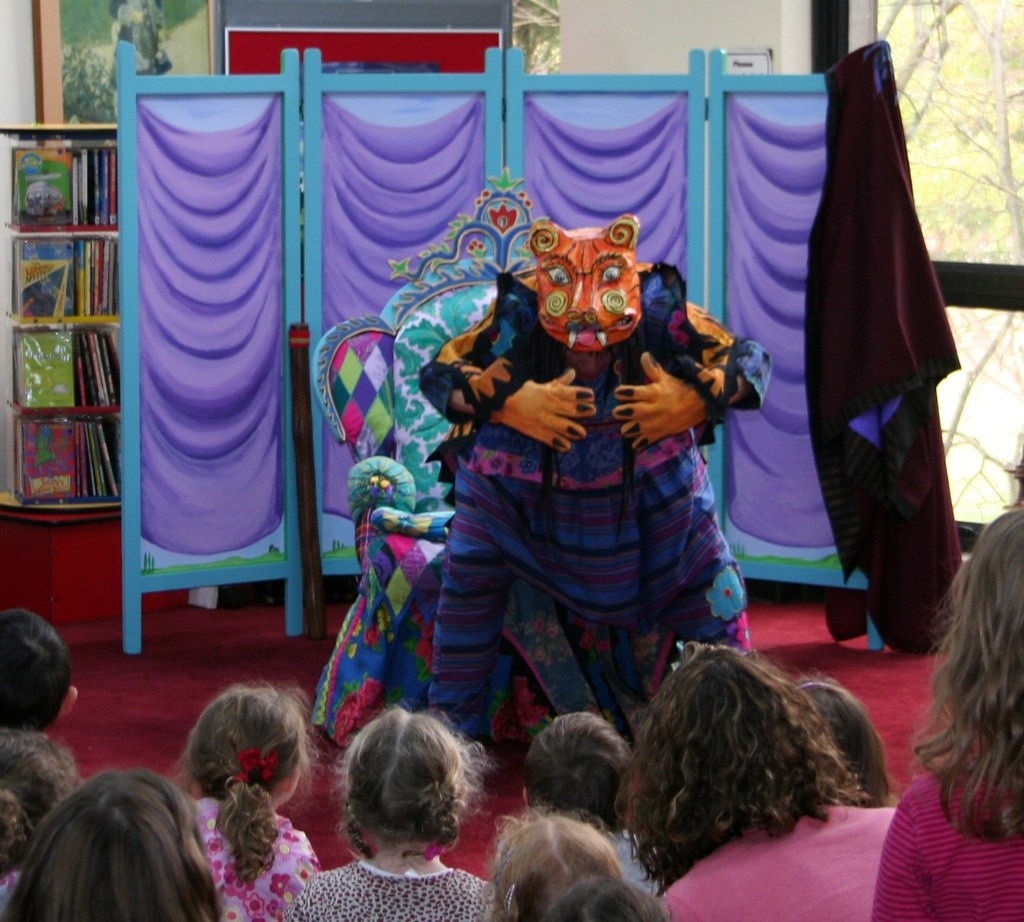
[488,816,670,922]
[0,768,224,922]
[794,677,899,808]
[178,687,322,922]
[871,510,1024,922]
[281,708,489,922]
[521,712,668,898]
[611,642,898,922]
[0,607,78,732]
[0,726,82,916]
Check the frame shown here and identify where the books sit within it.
[11,147,121,496]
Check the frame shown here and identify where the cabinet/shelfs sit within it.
[0,123,122,509]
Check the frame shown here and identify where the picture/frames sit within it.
[224,26,501,75]
[30,0,216,147]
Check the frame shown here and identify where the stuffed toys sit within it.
[416,213,771,748]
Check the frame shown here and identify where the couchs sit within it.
[306,165,752,752]
